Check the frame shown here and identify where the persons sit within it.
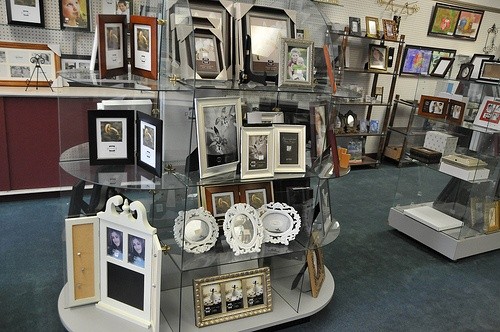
[204,288,221,305]
[107,230,123,261]
[62,0,81,27]
[107,28,119,49]
[452,105,460,118]
[225,284,242,302]
[247,280,263,297]
[288,48,306,81]
[438,103,443,113]
[117,0,129,22]
[315,107,325,155]
[372,48,384,65]
[431,102,440,113]
[137,29,149,52]
[289,57,306,81]
[128,235,145,268]
[482,104,500,121]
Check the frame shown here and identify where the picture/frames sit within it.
[2,2,499,326]
